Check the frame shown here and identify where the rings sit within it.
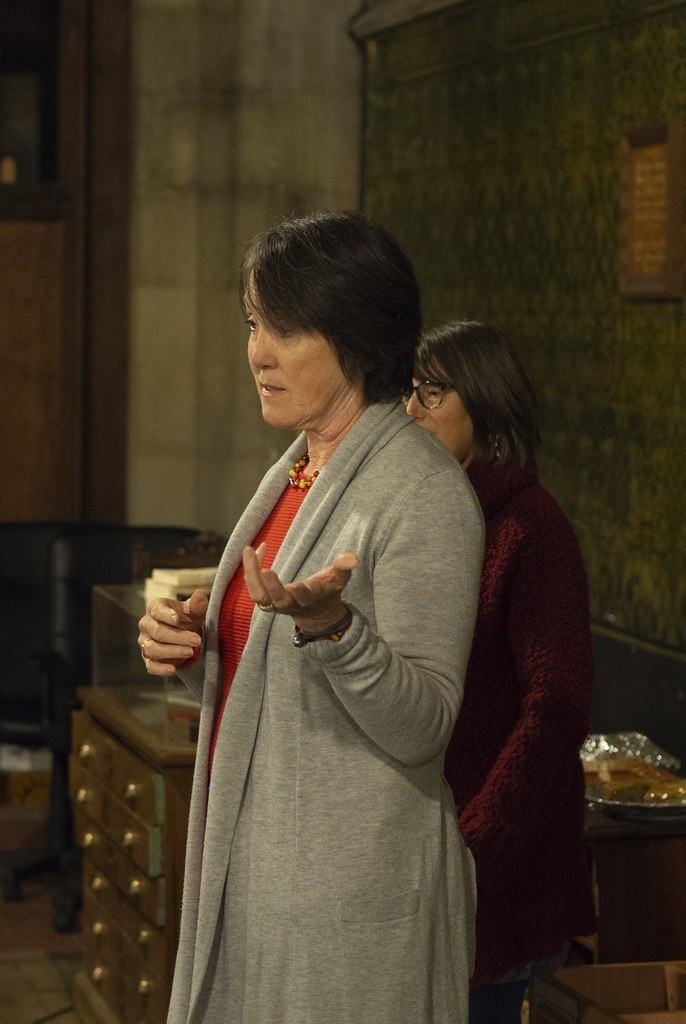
[259,603,273,612]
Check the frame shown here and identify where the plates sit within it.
[583,784,686,821]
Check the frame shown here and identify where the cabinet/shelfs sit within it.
[70,685,203,1024]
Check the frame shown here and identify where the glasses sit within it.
[401,378,454,410]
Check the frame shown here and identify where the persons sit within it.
[135,209,485,1024]
[402,321,600,1024]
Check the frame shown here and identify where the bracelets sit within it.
[293,606,352,647]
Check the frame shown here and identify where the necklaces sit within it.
[288,451,319,489]
